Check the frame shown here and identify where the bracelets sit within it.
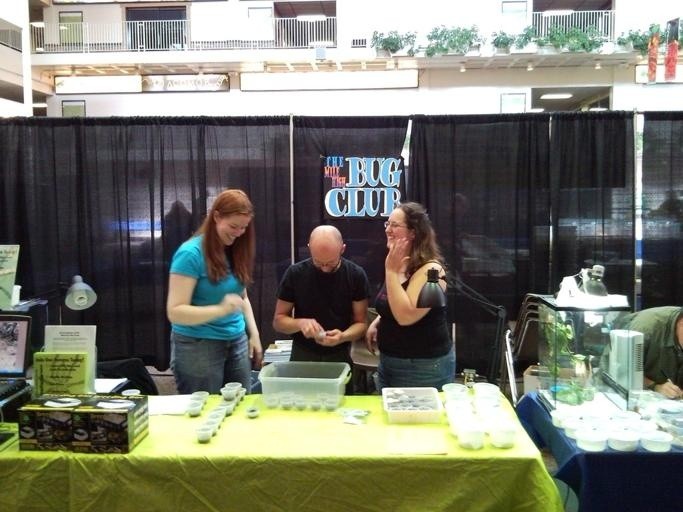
[647,381,655,391]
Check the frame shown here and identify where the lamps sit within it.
[58,275,98,325]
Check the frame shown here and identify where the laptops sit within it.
[0,315,32,401]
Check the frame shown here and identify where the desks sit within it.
[0,393,565,512]
[514,389,683,512]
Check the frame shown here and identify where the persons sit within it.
[166,190,265,394]
[272,224,370,395]
[366,202,457,393]
[600,305,683,400]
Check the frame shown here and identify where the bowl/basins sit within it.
[548,407,672,454]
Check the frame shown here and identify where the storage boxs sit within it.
[258,361,352,412]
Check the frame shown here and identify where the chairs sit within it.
[501,286,610,402]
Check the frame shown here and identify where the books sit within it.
[263,339,292,362]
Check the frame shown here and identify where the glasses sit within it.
[384,221,408,230]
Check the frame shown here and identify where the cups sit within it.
[186,383,258,443]
[266,393,339,411]
[442,382,515,448]
